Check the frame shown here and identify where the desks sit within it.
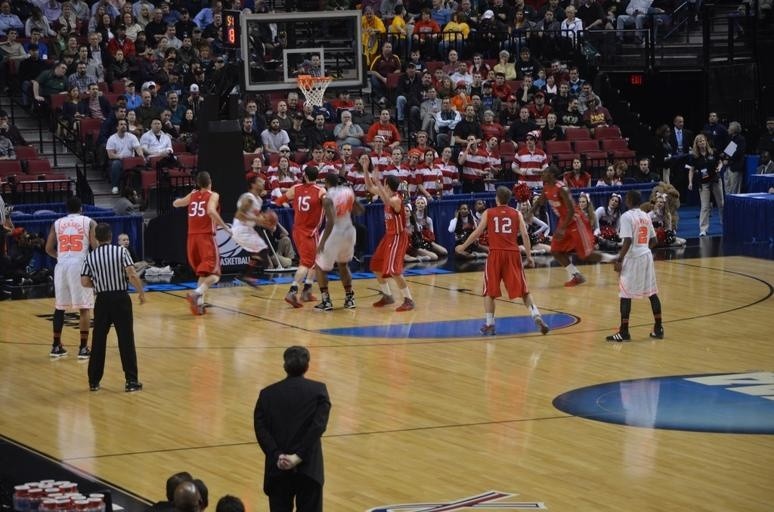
[746,173,774,192]
[722,192,774,260]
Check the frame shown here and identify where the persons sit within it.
[312,173,364,311]
[669,116,691,154]
[606,190,665,341]
[359,155,416,312]
[722,120,746,195]
[616,3,653,44]
[2,2,271,198]
[276,167,328,309]
[455,186,550,337]
[403,195,448,261]
[244,89,460,204]
[117,233,129,248]
[2,118,18,146]
[563,158,592,188]
[1,198,33,269]
[2,128,17,160]
[45,196,100,359]
[596,164,623,186]
[632,158,661,184]
[448,181,687,258]
[231,172,276,291]
[522,165,618,286]
[253,345,332,512]
[334,2,617,145]
[173,171,234,315]
[655,124,675,160]
[512,131,549,189]
[80,223,146,391]
[703,112,730,154]
[685,134,724,236]
[147,471,245,512]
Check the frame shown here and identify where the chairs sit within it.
[0,0,751,203]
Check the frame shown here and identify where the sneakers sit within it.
[78,346,91,358]
[50,343,67,357]
[125,379,142,391]
[89,383,102,391]
